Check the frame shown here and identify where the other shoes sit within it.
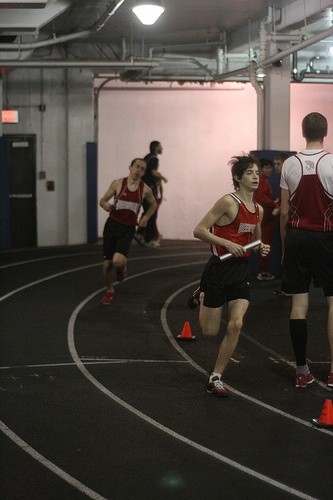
[134,233,148,247]
[148,241,161,248]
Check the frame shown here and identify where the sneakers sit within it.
[101,290,114,304]
[327,373,333,386]
[296,372,314,388]
[188,287,201,309]
[116,267,127,283]
[207,380,229,397]
[257,273,275,280]
[280,289,293,296]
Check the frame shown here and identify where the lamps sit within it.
[131,0,166,26]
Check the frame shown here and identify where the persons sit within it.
[192,156,271,397]
[100,158,158,304]
[254,158,275,281]
[268,153,289,214]
[278,112,333,391]
[136,141,167,249]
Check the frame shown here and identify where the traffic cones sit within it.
[312,400,333,428]
[176,322,196,341]
